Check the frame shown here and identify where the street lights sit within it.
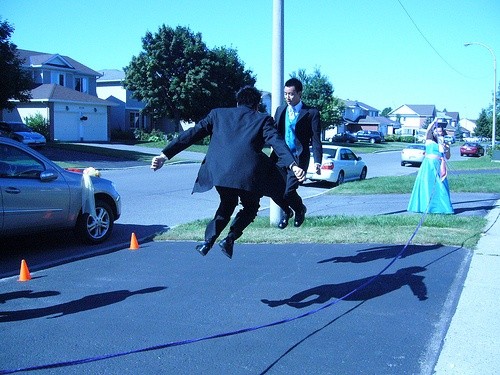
[463,42,496,150]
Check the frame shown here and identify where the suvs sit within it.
[353,130,381,143]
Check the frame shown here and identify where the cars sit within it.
[0,137,123,243]
[299,145,368,187]
[332,133,354,143]
[401,143,426,167]
[444,136,455,144]
[460,142,485,156]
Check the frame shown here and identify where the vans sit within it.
[0,121,47,146]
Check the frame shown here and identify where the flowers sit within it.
[61,167,100,218]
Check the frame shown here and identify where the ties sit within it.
[290,106,296,122]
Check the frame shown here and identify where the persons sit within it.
[405,118,455,215]
[259,79,322,230]
[148,86,306,260]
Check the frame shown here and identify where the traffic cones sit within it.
[128,232,139,249]
[16,259,32,281]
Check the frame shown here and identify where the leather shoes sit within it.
[278,208,294,229]
[196,243,211,257]
[294,204,307,227]
[218,238,234,259]
